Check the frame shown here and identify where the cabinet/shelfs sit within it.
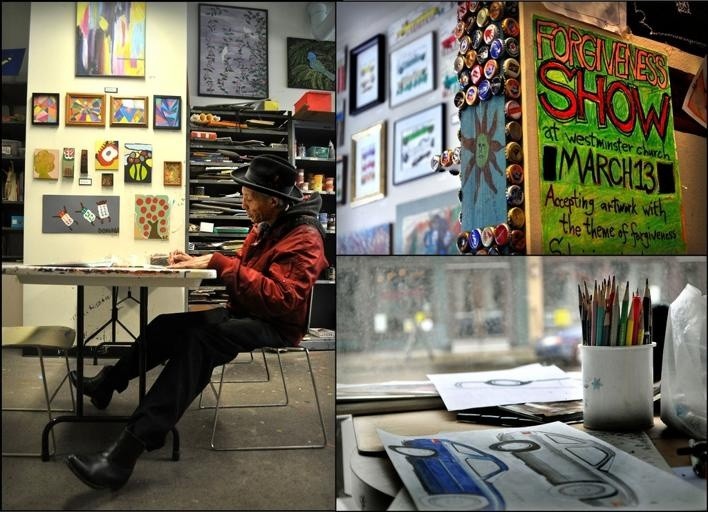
[187,110,336,305]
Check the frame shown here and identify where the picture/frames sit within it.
[66,92,106,126]
[198,3,270,100]
[32,93,59,125]
[110,95,148,127]
[387,30,437,108]
[348,120,386,209]
[349,33,385,116]
[153,95,181,129]
[392,103,446,185]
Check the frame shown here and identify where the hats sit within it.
[230,154,306,202]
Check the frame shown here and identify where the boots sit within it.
[65,426,145,492]
[69,364,128,410]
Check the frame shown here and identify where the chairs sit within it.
[2,326,75,456]
[199,285,327,451]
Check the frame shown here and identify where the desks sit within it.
[334,254,707,511]
[0,263,219,462]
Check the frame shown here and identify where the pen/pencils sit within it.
[457,413,544,426]
[170,249,178,266]
[578,275,653,346]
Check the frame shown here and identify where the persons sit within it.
[402,284,437,360]
[62,153,330,491]
